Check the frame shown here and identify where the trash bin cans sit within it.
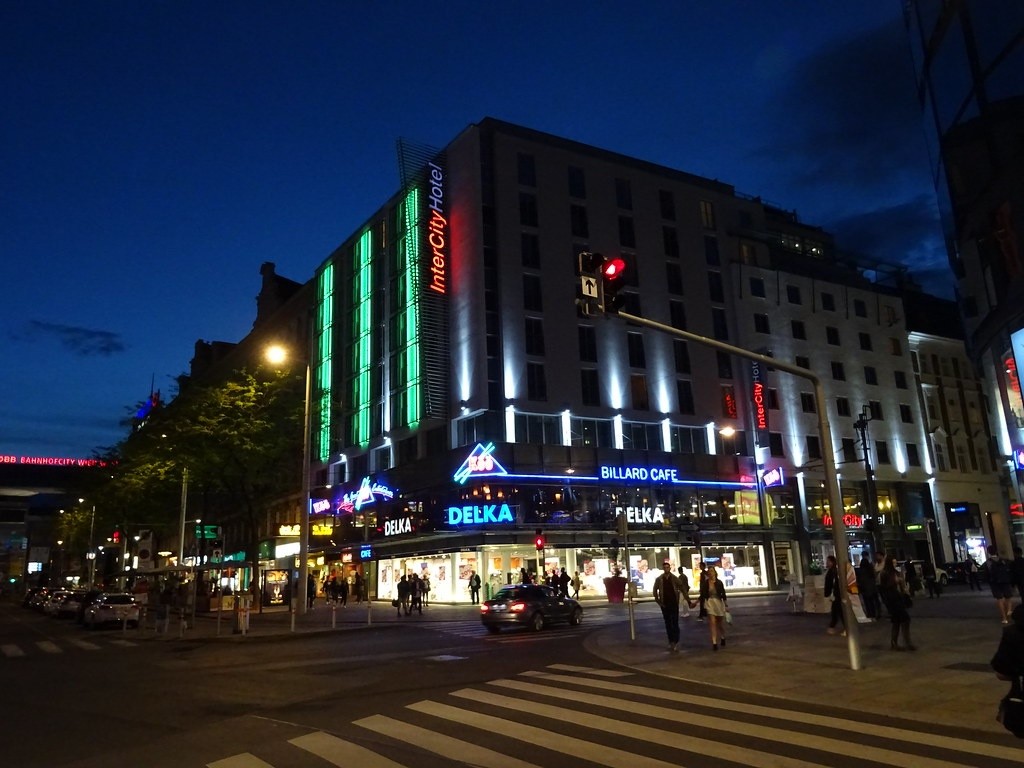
[233,595,250,633]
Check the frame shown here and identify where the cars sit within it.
[23,587,89,620]
[480,584,584,632]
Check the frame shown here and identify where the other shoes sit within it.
[828,627,834,634]
[891,645,905,652]
[1001,620,1008,623]
[713,643,717,651]
[1008,611,1013,615]
[721,636,725,646]
[908,645,917,651]
[669,642,679,654]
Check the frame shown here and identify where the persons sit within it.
[468,570,481,605]
[824,555,846,635]
[653,563,693,651]
[306,572,364,609]
[546,568,580,601]
[678,567,690,617]
[697,563,708,622]
[689,566,729,650]
[397,573,431,618]
[521,568,531,584]
[876,553,916,651]
[965,546,1024,739]
[849,551,937,620]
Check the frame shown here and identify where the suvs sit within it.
[895,560,949,587]
[85,592,139,628]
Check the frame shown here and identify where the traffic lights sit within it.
[536,534,544,551]
[601,257,626,321]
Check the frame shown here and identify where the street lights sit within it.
[78,497,95,583]
[262,345,311,615]
[161,433,187,565]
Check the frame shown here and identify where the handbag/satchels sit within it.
[725,610,733,626]
[900,593,913,609]
